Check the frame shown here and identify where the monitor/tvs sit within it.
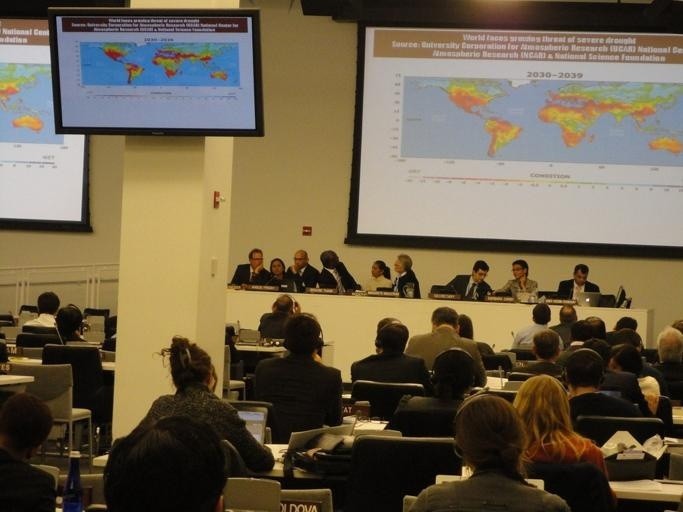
[614,286,625,308]
[48,6,264,137]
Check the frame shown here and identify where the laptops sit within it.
[576,291,601,307]
[436,475,545,491]
[233,406,268,448]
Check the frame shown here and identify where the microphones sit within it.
[492,344,495,349]
[499,365,504,390]
[512,331,515,338]
[350,410,362,435]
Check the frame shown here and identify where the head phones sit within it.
[561,348,607,386]
[453,394,497,459]
[294,313,324,349]
[287,294,296,314]
[375,320,399,346]
[430,347,476,386]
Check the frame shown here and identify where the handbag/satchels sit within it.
[285,428,402,471]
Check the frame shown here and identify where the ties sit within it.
[467,283,477,298]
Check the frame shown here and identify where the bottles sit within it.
[62,450,84,510]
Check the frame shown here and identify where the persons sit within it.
[1,392,62,510]
[23,290,117,438]
[227,249,599,302]
[405,392,572,512]
[140,296,683,475]
[510,373,618,509]
[100,416,227,512]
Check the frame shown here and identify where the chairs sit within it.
[0,281,681,511]
